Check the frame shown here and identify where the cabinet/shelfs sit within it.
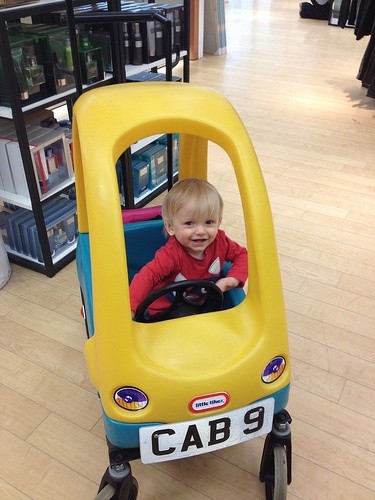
[1,0,190,279]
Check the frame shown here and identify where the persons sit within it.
[128,177,248,322]
